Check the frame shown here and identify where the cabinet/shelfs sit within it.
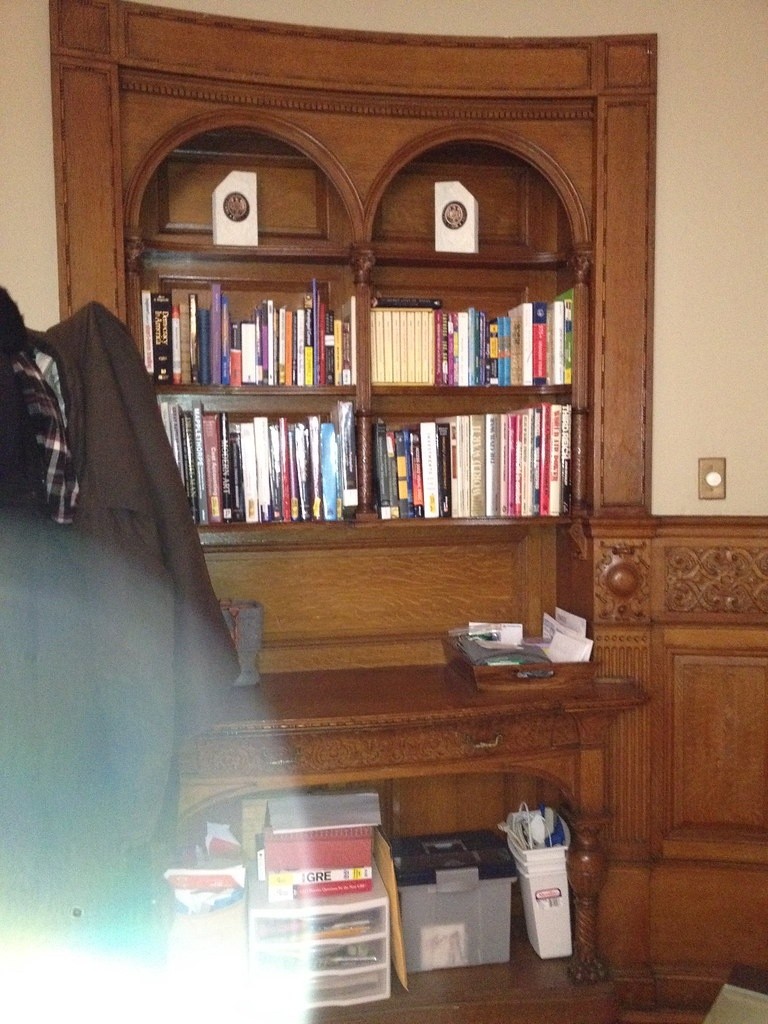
[46,2,662,1024]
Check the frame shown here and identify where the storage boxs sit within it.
[167,808,572,1009]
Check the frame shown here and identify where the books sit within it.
[372,402,572,519]
[160,398,358,525]
[370,288,575,386]
[140,276,356,386]
